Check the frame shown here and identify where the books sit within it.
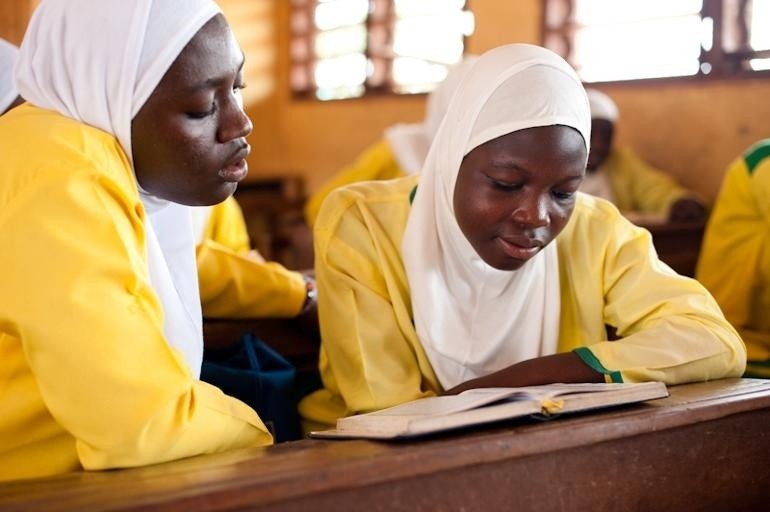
[308,381,670,441]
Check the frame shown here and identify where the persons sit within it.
[694,138,770,378]
[576,88,708,222]
[0,0,318,483]
[302,123,427,226]
[297,42,747,441]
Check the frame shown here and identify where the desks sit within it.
[0,170,770,511]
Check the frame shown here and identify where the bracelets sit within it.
[303,279,315,310]
